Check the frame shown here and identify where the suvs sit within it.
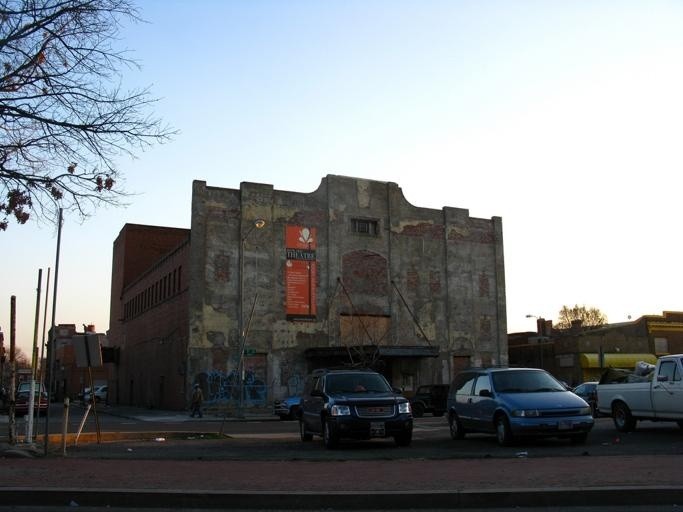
[298,367,412,449]
[409,383,449,417]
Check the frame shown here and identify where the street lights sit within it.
[240,219,265,381]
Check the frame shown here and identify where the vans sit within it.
[445,364,593,446]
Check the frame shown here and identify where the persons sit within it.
[188,383,203,418]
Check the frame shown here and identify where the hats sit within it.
[193,384,199,387]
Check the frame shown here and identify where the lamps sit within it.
[243,219,266,241]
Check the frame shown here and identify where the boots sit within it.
[189,413,203,418]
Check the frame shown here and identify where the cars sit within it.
[572,381,600,418]
[84,385,107,404]
[15,380,48,417]
[273,395,301,420]
[78,386,98,399]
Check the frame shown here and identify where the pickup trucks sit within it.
[595,354,682,433]
